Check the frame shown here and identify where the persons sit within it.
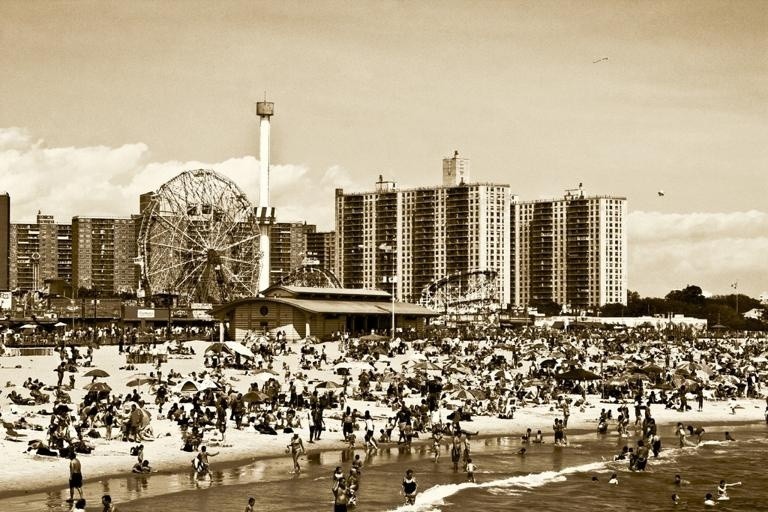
[2,326,766,511]
